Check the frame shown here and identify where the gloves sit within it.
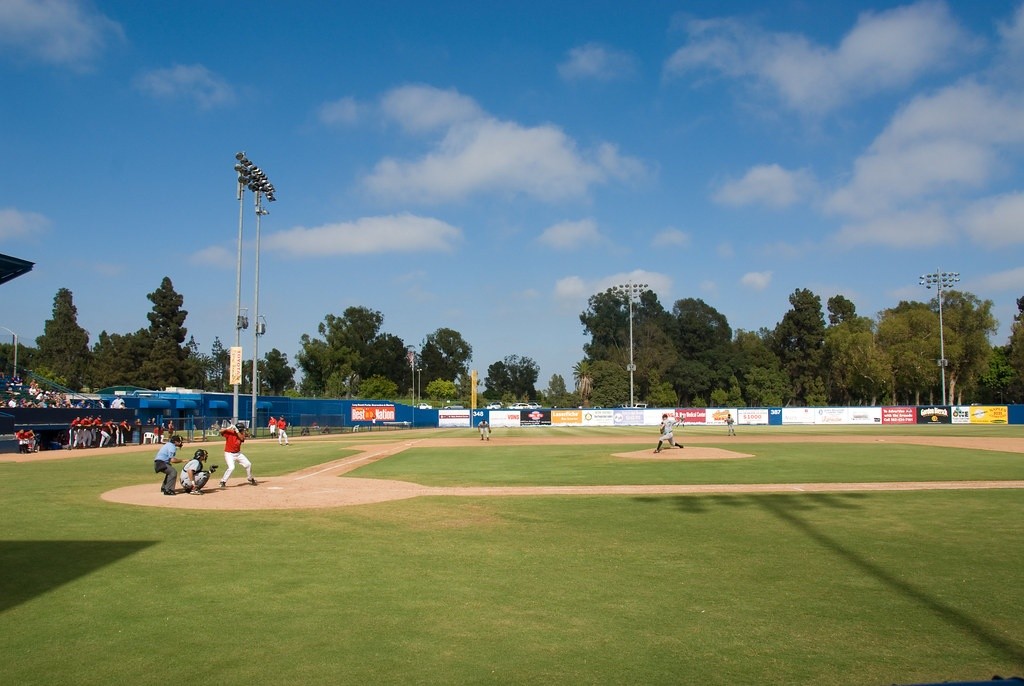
[226,423,239,433]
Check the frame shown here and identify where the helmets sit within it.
[194,449,205,459]
[172,436,180,442]
[235,423,245,431]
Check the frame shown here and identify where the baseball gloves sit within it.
[209,465,220,473]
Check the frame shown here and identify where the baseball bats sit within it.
[226,417,236,429]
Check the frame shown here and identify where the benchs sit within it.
[0,375,57,407]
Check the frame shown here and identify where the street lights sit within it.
[229,149,278,443]
[612,280,649,407]
[416,368,422,406]
[919,267,960,407]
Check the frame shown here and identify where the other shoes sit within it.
[161,486,166,492]
[219,482,225,489]
[247,478,257,486]
[191,489,204,495]
[164,490,176,495]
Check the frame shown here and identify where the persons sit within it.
[478,418,491,441]
[154,436,191,495]
[180,449,219,495]
[725,413,736,436]
[654,414,684,453]
[0,373,376,454]
[219,423,257,487]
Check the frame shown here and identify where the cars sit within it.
[444,406,464,409]
[414,402,432,410]
[618,404,648,409]
[486,401,502,409]
[528,403,543,409]
[507,403,531,410]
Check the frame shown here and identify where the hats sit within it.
[98,415,102,418]
[90,414,93,418]
[280,415,284,418]
[85,416,89,419]
[124,419,128,422]
